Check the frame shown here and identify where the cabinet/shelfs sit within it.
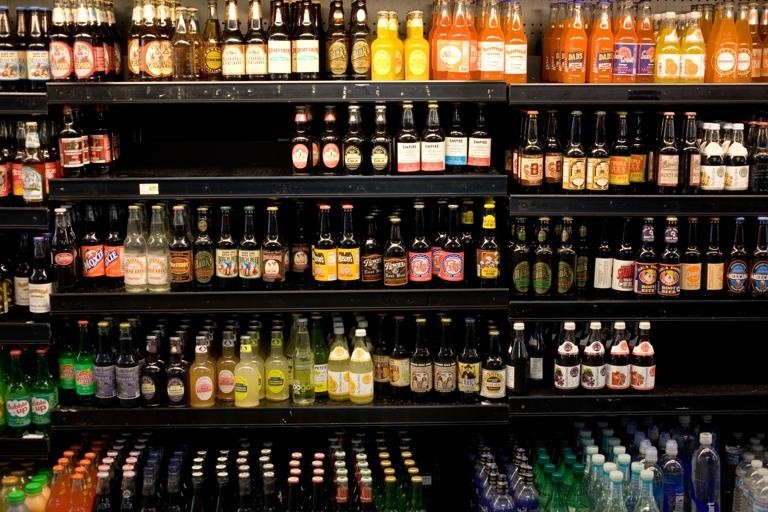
[0,83,768,460]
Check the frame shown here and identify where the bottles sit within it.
[0,1,768,94]
[0,314,652,432]
[0,411,768,510]
[0,204,768,327]
[0,103,123,206]
[288,101,768,192]
